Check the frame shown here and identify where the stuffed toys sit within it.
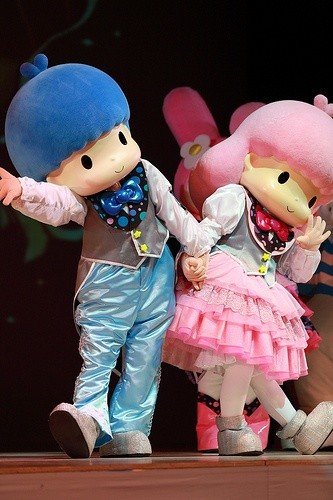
[162,87,270,451]
[161,94,333,456]
[0,54,211,458]
